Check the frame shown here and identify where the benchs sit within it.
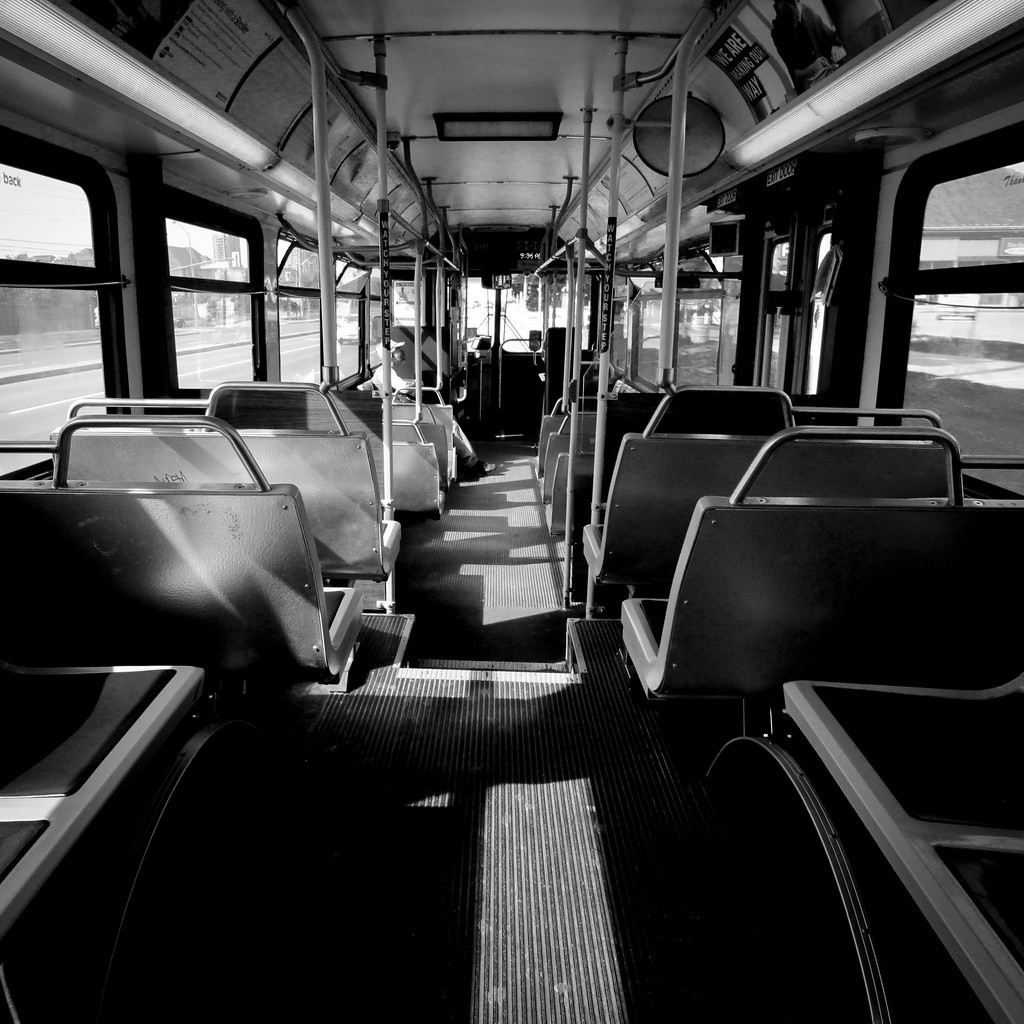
[0,379,453,1024]
[539,391,1024,1024]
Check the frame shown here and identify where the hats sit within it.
[377,339,405,359]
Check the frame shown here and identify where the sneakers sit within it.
[475,459,497,476]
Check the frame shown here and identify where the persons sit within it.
[375,340,497,475]
[496,280,509,288]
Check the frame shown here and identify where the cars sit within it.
[337,321,378,346]
[173,317,184,328]
